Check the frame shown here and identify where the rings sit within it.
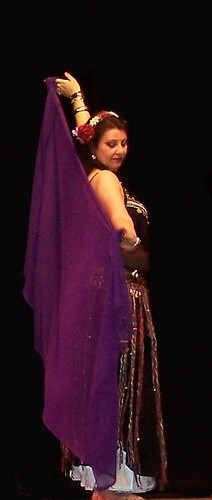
[58,91,61,95]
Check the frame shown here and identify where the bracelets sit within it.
[69,90,86,104]
[73,106,89,115]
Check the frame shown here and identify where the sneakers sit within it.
[78,462,86,488]
[83,446,154,494]
[69,465,82,482]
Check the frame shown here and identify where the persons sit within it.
[44,70,157,493]
